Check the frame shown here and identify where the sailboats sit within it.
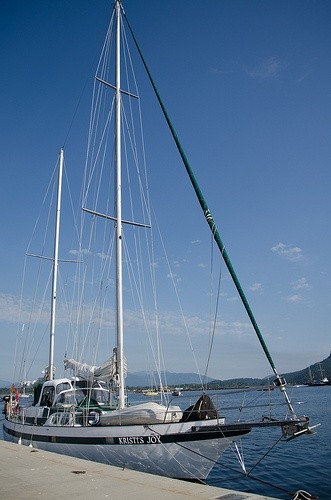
[1,1,322,482]
